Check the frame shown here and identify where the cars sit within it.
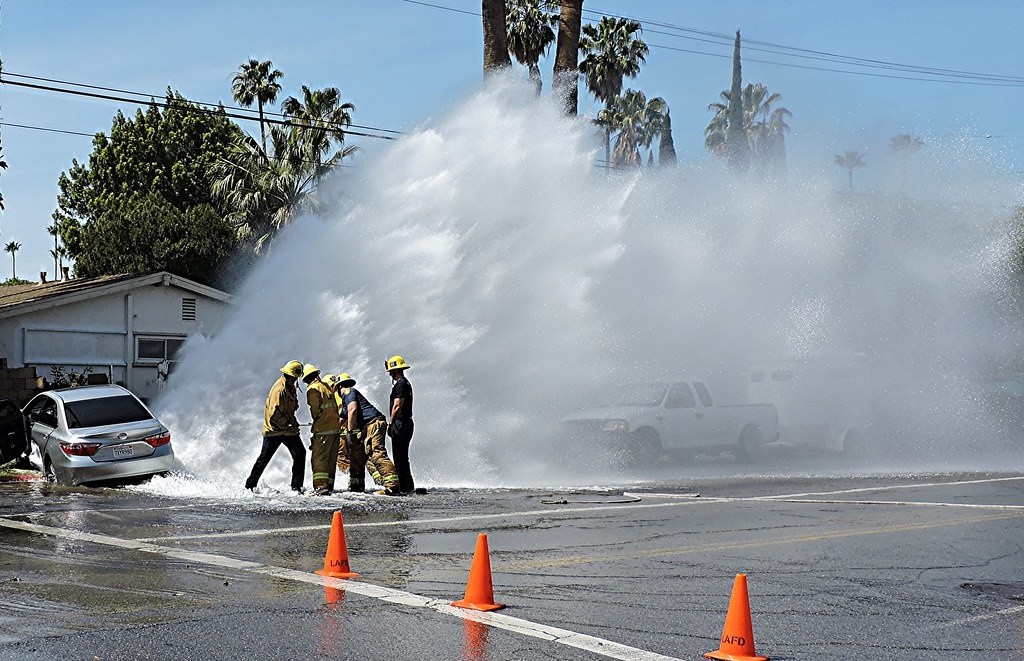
[0,383,174,488]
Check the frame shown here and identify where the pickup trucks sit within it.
[563,378,778,465]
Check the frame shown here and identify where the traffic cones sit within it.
[450,531,507,611]
[314,511,360,578]
[702,571,769,661]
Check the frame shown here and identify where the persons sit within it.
[240,360,310,494]
[302,361,399,496]
[385,356,414,495]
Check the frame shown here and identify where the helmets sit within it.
[333,373,356,391]
[322,374,338,387]
[385,355,411,372]
[302,363,321,383]
[280,360,303,378]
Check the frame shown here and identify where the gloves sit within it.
[288,417,300,431]
[346,431,355,446]
[388,423,392,438]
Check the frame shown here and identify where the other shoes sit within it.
[244,486,258,494]
[399,488,412,496]
[364,484,385,493]
[292,486,304,494]
[314,487,331,496]
[372,485,400,495]
